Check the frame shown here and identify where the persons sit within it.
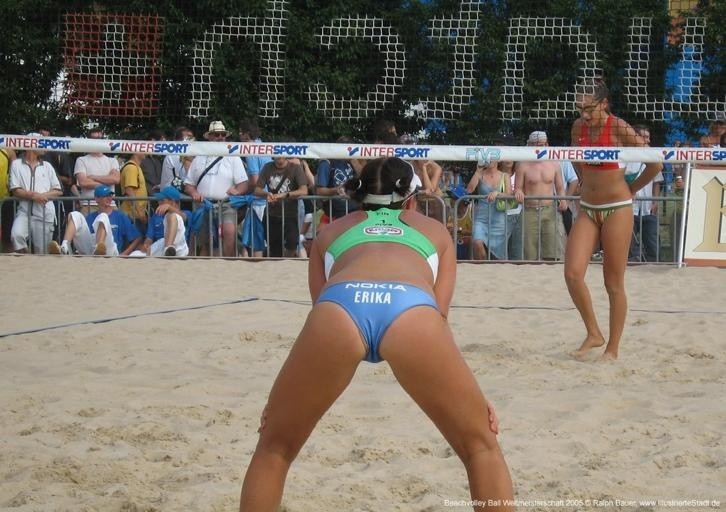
[239,157,516,512]
[564,76,663,363]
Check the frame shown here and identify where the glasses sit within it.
[575,98,605,113]
[210,132,227,137]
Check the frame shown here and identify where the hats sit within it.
[492,133,514,146]
[711,110,726,124]
[202,120,232,139]
[360,156,423,207]
[152,185,181,202]
[445,183,470,205]
[94,185,115,197]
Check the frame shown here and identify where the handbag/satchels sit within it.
[495,193,519,212]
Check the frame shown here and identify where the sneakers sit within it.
[47,240,70,255]
[162,245,177,256]
[91,241,107,255]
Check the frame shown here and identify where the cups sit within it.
[676,175,684,181]
